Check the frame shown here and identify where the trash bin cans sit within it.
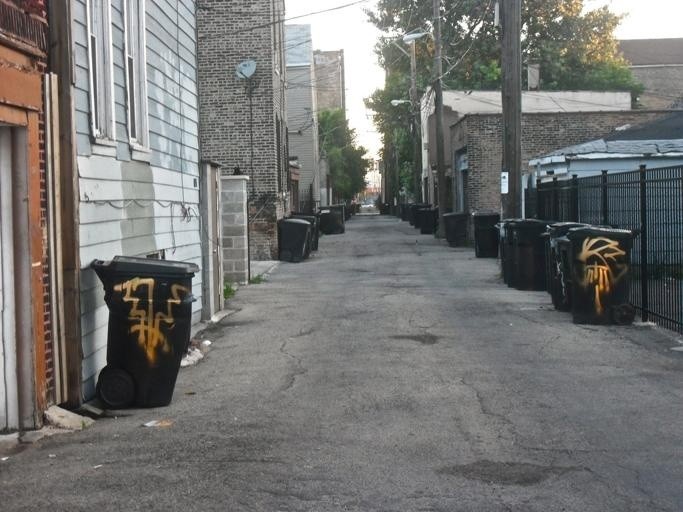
[549,222,638,322]
[387,204,411,221]
[419,208,436,234]
[90,255,201,409]
[409,202,432,229]
[501,218,548,290]
[277,215,319,262]
[443,212,470,247]
[473,212,500,258]
[320,204,346,235]
